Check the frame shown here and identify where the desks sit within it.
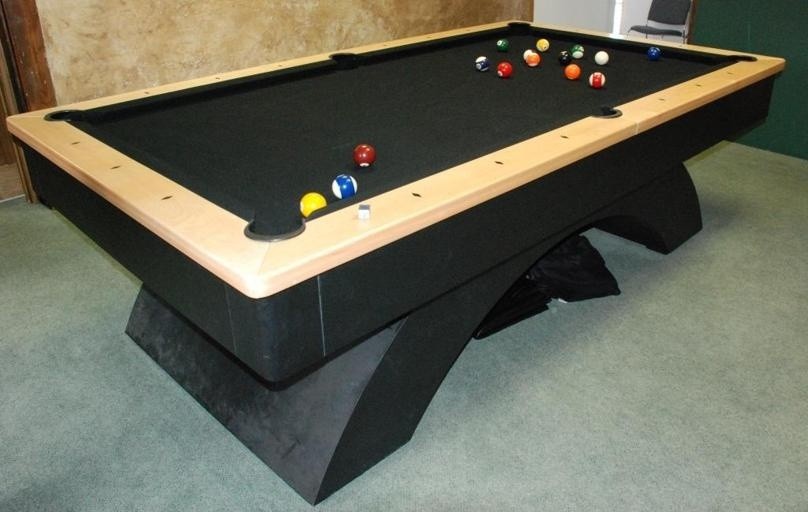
[5,19,786,507]
